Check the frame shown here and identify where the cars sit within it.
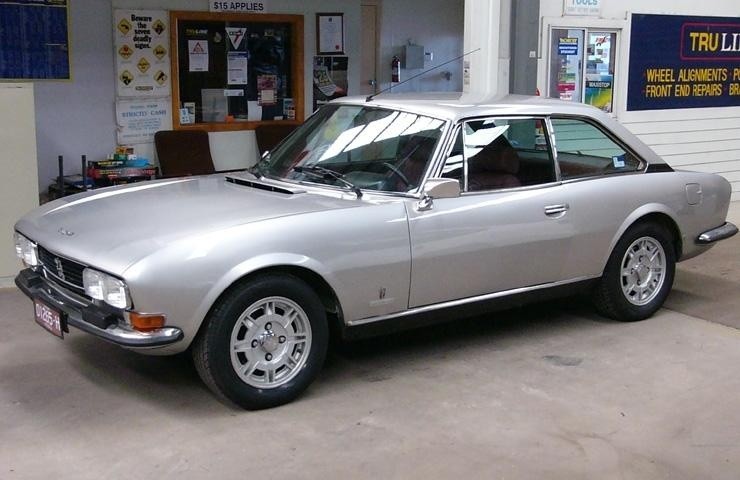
[11,84,739,410]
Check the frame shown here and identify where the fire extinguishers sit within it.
[391,56,401,82]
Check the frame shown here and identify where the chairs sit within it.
[155,129,216,179]
[394,135,437,194]
[468,122,523,191]
[254,124,309,164]
